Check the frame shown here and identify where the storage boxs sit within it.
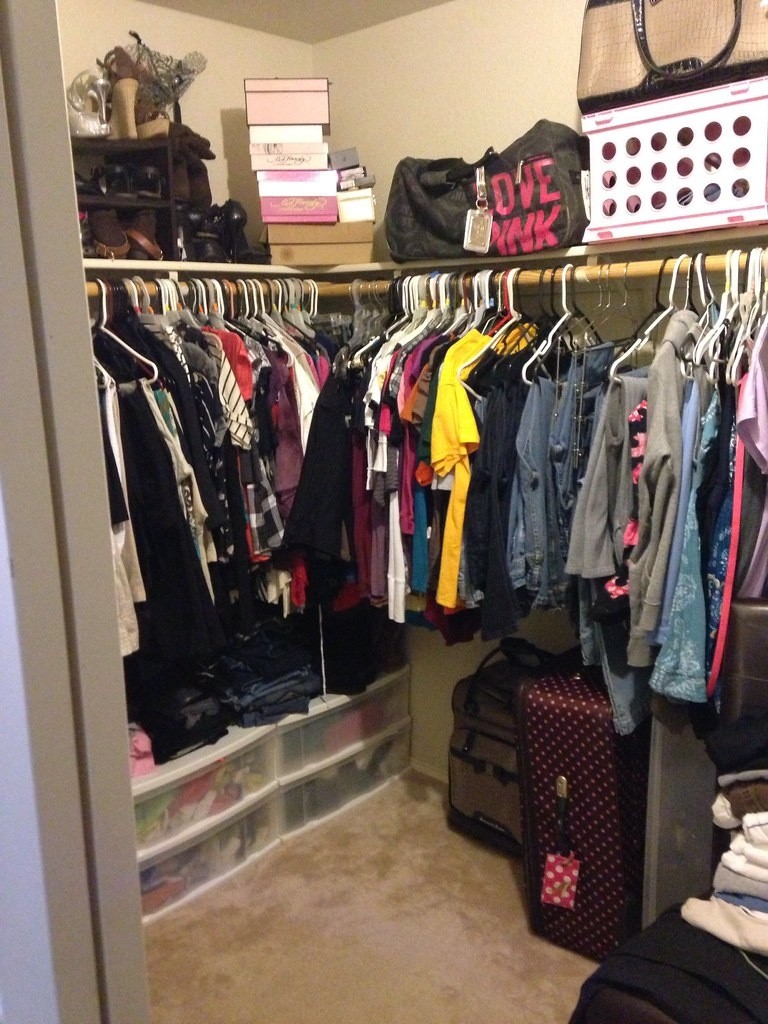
[131,662,414,930]
[581,77,768,245]
[244,77,375,266]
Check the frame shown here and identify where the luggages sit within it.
[447,642,541,850]
[516,647,646,963]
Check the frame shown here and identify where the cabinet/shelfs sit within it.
[72,135,216,261]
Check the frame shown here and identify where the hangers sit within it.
[90,275,318,389]
[331,246,768,403]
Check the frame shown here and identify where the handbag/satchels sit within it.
[577,1,768,115]
[385,119,591,263]
